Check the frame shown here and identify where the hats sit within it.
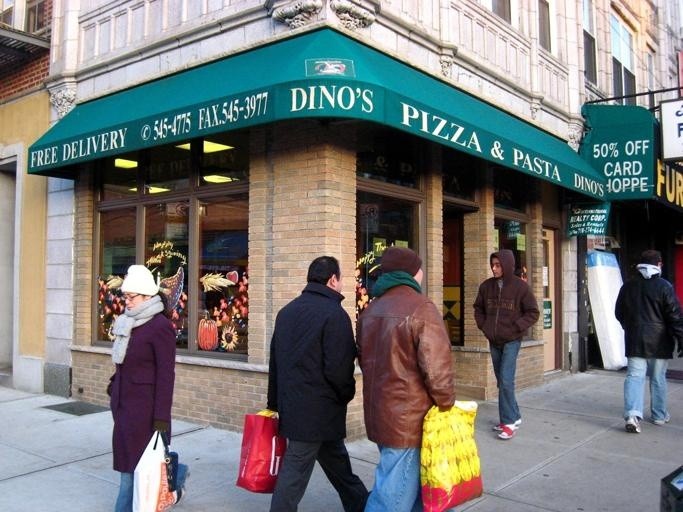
[380,244,422,275]
[122,264,159,296]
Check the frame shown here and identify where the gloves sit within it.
[107,382,114,397]
[154,419,170,433]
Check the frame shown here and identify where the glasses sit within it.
[121,295,140,302]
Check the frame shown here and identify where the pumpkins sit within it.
[197,312,218,351]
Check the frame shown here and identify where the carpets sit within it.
[42,401,111,416]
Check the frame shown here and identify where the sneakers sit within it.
[499,427,519,440]
[494,421,521,431]
[651,412,670,425]
[174,466,188,506]
[625,415,641,432]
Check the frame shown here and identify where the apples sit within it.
[227,271,238,283]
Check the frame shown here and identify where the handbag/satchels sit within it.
[236,414,288,495]
[165,452,178,492]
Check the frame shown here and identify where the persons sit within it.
[615,249,682,434]
[106,262,187,512]
[263,254,371,511]
[354,245,456,512]
[471,248,539,440]
